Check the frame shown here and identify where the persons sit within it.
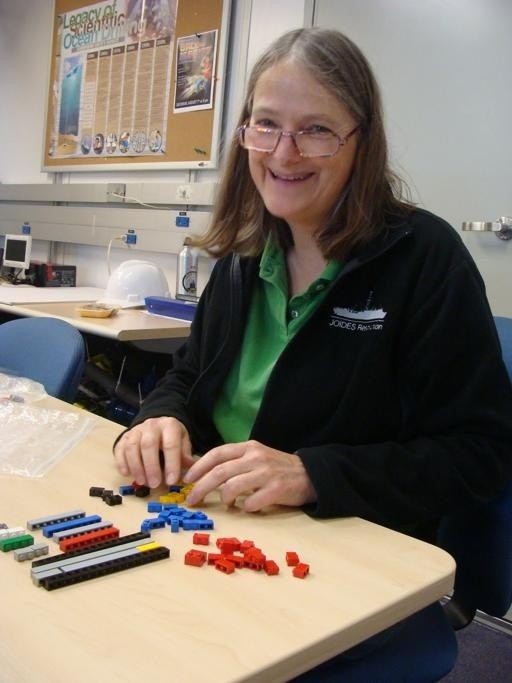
[112,24,512,682]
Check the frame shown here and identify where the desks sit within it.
[1,281,192,358]
[0,372,456,681]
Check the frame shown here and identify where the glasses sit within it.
[235,116,361,161]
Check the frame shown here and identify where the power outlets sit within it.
[124,233,136,244]
[176,216,189,226]
[22,225,30,233]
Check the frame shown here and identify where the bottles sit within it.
[175,236,200,301]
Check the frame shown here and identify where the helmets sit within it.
[97,260,172,310]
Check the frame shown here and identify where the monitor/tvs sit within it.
[3,234,32,280]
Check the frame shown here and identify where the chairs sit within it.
[0,316,91,400]
[286,318,511,681]
